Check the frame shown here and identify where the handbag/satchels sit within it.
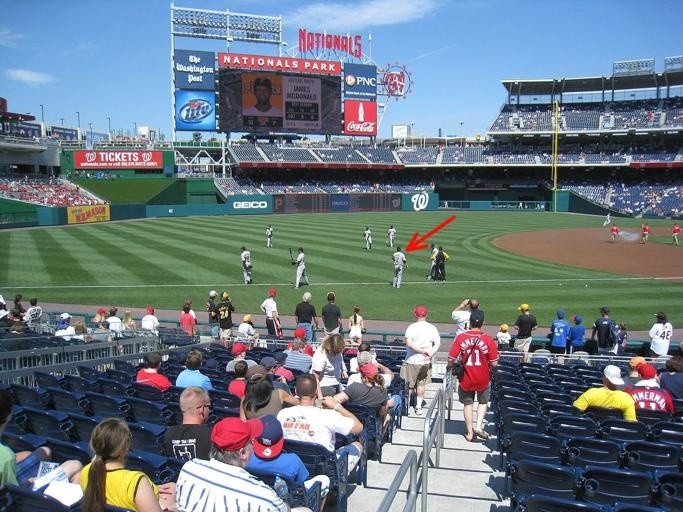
[453,361,464,379]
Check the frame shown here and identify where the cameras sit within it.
[467,299,470,305]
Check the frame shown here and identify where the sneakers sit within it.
[475,429,488,439]
[415,406,424,414]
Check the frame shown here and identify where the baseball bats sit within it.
[288,247,295,262]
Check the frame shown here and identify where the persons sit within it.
[602,213,610,227]
[81,417,178,511]
[239,246,254,283]
[265,228,270,247]
[1,288,682,479]
[640,223,650,243]
[362,232,371,249]
[242,414,329,511]
[426,243,441,277]
[426,246,448,282]
[365,227,370,233]
[392,246,408,288]
[671,222,681,247]
[611,224,619,241]
[0,387,83,496]
[293,246,309,288]
[1,99,682,215]
[176,418,314,511]
[243,77,281,117]
[268,225,273,247]
[387,225,395,247]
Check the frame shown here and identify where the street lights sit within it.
[662,54,683,102]
[170,2,283,57]
[411,122,416,147]
[39,104,137,143]
[611,57,659,112]
[459,121,464,146]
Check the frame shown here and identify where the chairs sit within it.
[485,352,681,512]
[1,339,417,512]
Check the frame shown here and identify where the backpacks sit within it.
[608,326,617,348]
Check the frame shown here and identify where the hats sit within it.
[572,314,582,322]
[518,303,529,310]
[558,310,564,318]
[604,356,656,385]
[471,310,484,324]
[415,305,426,317]
[501,323,508,331]
[209,288,379,383]
[600,306,609,313]
[60,313,71,319]
[210,414,283,459]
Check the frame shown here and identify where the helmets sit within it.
[99,308,106,314]
[147,307,154,314]
